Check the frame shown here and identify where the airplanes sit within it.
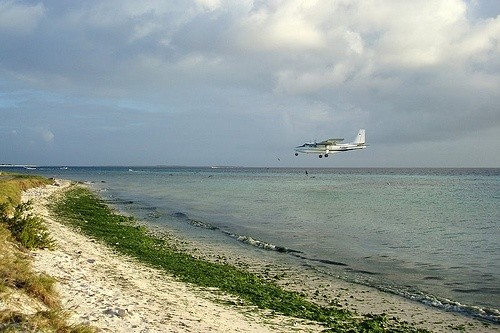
[293,128,372,158]
